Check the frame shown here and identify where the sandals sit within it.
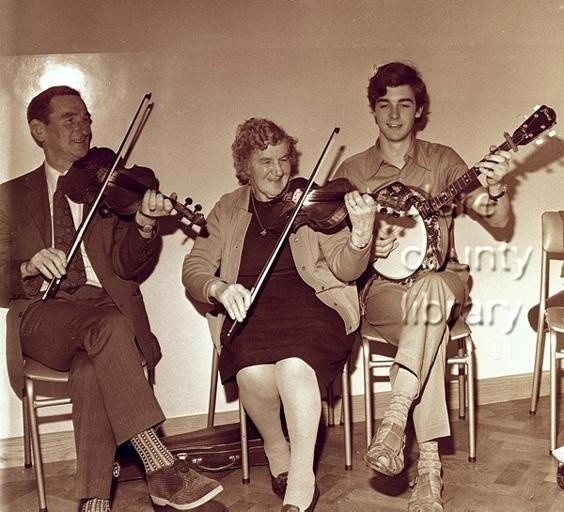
[408,472,445,511]
[361,420,410,477]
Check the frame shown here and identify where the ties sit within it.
[53,176,87,295]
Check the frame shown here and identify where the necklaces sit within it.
[244,188,298,239]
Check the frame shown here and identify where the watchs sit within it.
[135,221,155,234]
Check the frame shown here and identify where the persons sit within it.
[1,84,225,511]
[329,61,512,512]
[181,117,385,511]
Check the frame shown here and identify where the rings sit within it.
[351,204,359,212]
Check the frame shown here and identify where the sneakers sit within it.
[146,455,223,508]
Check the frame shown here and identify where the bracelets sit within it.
[482,184,512,202]
[345,234,372,250]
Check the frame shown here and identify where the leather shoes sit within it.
[268,450,320,512]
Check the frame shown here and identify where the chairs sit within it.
[206,344,353,484]
[341,318,476,463]
[529,208,564,455]
[21,355,149,511]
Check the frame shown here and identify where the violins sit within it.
[62,148,207,228]
[265,178,410,236]
